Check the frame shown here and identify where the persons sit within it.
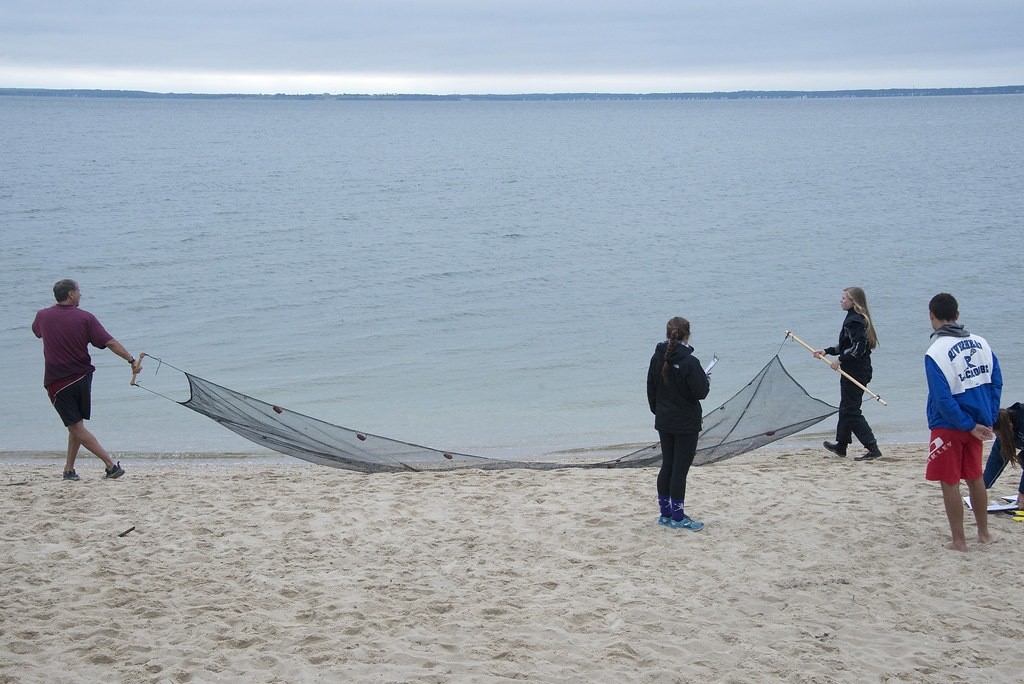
[924,293,1002,553]
[813,287,882,461]
[31,279,142,480]
[982,402,1024,521]
[647,317,711,532]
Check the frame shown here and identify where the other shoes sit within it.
[823,441,846,457]
[854,450,882,461]
[104,461,125,479]
[63,469,79,481]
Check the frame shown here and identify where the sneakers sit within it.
[669,514,705,532]
[659,515,672,528]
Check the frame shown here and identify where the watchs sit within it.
[128,356,136,363]
